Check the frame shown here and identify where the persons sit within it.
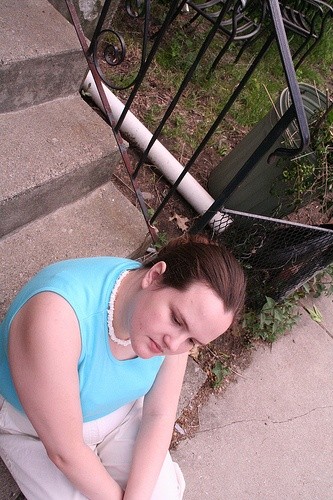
[0,234,247,500]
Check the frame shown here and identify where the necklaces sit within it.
[106,270,131,347]
